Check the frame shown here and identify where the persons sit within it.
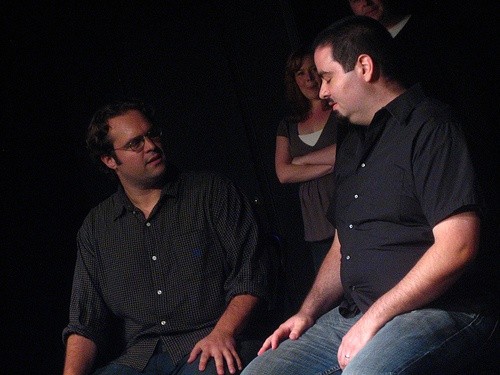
[241,15,499,375]
[274,41,338,277]
[348,0,441,79]
[62,100,278,375]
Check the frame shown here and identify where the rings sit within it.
[345,355,350,358]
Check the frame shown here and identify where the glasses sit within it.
[113,123,163,151]
